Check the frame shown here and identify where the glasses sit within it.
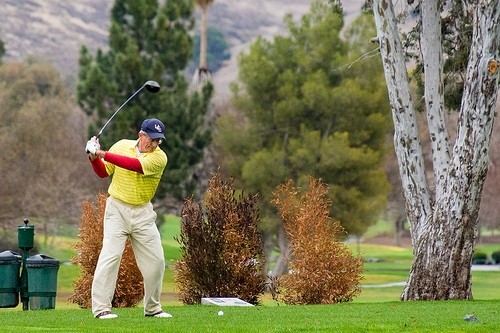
[142,133,162,145]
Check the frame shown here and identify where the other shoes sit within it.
[96,311,118,318]
[154,312,172,317]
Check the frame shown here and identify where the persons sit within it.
[86,118,172,319]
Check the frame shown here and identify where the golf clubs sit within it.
[217,311,224,317]
[86,80,161,154]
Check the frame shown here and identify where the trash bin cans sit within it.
[0,249,62,311]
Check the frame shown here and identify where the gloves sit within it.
[91,135,100,150]
[85,139,99,156]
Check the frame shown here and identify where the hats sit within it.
[141,118,167,140]
